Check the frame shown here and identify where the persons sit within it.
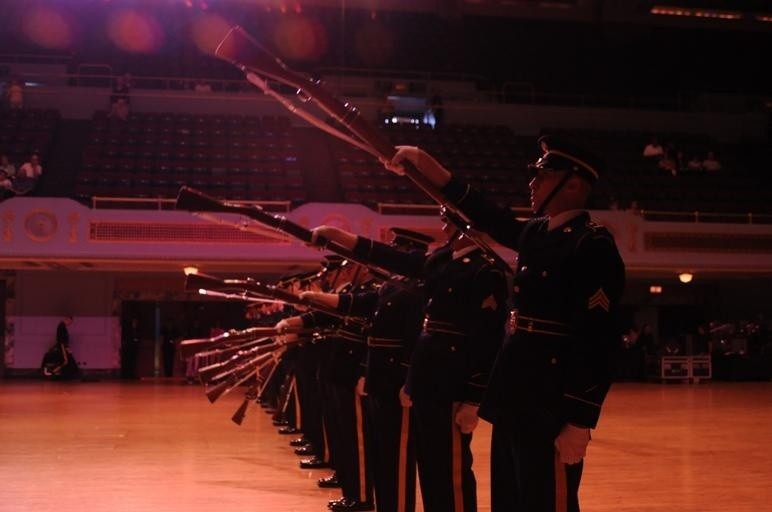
[644,138,663,155]
[635,325,657,381]
[432,89,443,128]
[6,81,23,110]
[159,322,176,377]
[195,81,211,92]
[127,316,139,378]
[676,150,723,171]
[0,152,43,199]
[106,73,136,123]
[53,312,73,380]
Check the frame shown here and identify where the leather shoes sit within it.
[259,403,298,435]
[289,440,375,512]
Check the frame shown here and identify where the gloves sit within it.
[356,376,366,395]
[456,405,480,437]
[556,424,591,466]
[398,387,413,407]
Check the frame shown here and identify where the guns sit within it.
[176,185,467,301]
[178,269,366,425]
[214,26,517,281]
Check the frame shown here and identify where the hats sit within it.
[390,227,436,252]
[277,254,344,291]
[525,133,609,184]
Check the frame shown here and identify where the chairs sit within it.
[0,108,772,227]
